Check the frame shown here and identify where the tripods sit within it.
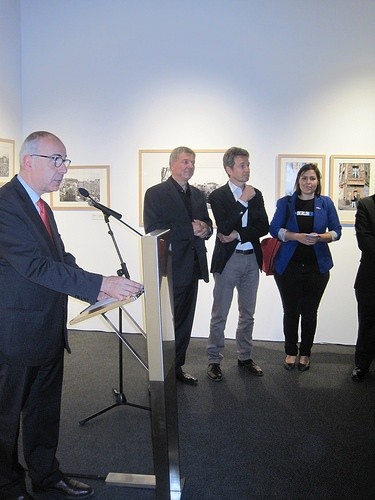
[78,213,151,424]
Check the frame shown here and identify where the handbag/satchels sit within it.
[259,238,282,276]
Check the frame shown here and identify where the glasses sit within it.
[28,154,70,168]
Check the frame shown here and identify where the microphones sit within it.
[86,197,122,219]
[78,188,143,236]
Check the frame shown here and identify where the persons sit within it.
[351,193,375,381]
[207,146,270,381]
[143,146,214,382]
[269,164,343,371]
[0,130,142,500]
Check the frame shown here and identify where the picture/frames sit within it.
[0,138,16,186]
[138,148,228,228]
[330,154,375,224]
[50,165,110,210]
[277,153,327,200]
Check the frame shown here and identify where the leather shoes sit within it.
[350,366,370,381]
[39,476,94,498]
[284,355,295,371]
[298,356,310,371]
[174,368,198,384]
[207,362,222,381]
[237,359,263,375]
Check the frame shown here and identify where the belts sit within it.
[233,249,255,255]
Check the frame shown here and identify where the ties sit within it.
[37,198,54,244]
